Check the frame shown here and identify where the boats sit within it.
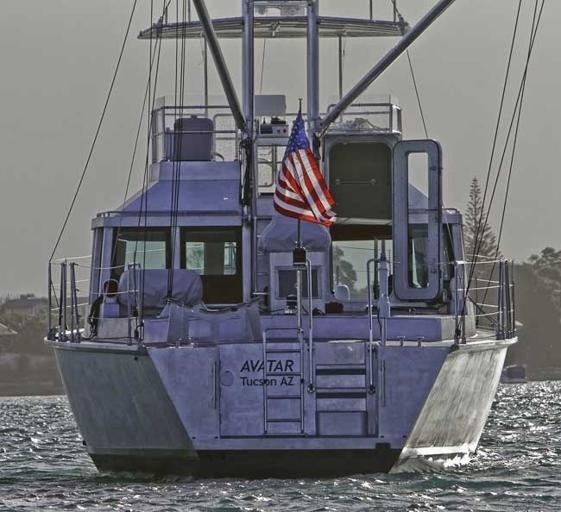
[46,0,549,478]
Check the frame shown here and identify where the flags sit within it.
[274,110,337,228]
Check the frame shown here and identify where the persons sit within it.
[87,278,121,333]
